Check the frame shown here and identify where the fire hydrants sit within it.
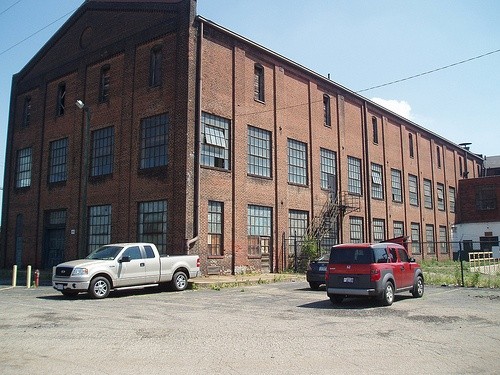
[34,269,40,287]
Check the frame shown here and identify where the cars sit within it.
[305,254,329,291]
[325,242,424,308]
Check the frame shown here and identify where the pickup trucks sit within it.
[52,242,200,299]
